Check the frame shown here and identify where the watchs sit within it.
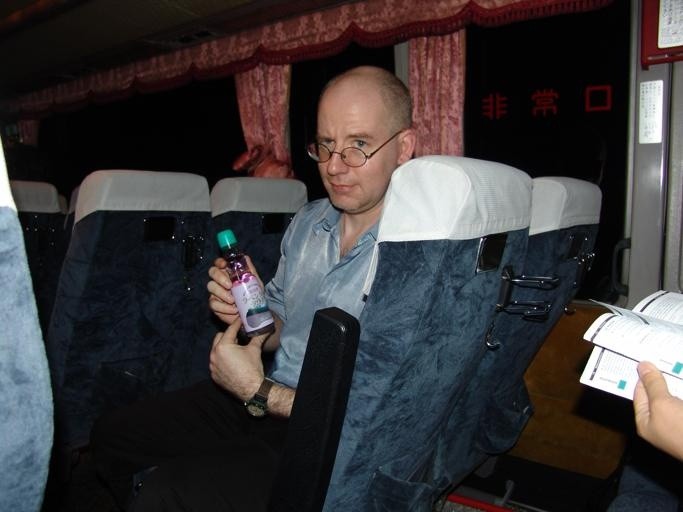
[245,378,275,418]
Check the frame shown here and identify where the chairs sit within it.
[1,142,311,512]
[416,176,601,501]
[269,156,530,502]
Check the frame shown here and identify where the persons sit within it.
[66,66,417,512]
[606,362,682,511]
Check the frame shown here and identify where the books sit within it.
[579,290,682,403]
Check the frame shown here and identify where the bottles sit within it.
[216,228,277,339]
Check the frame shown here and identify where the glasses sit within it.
[307,131,401,168]
